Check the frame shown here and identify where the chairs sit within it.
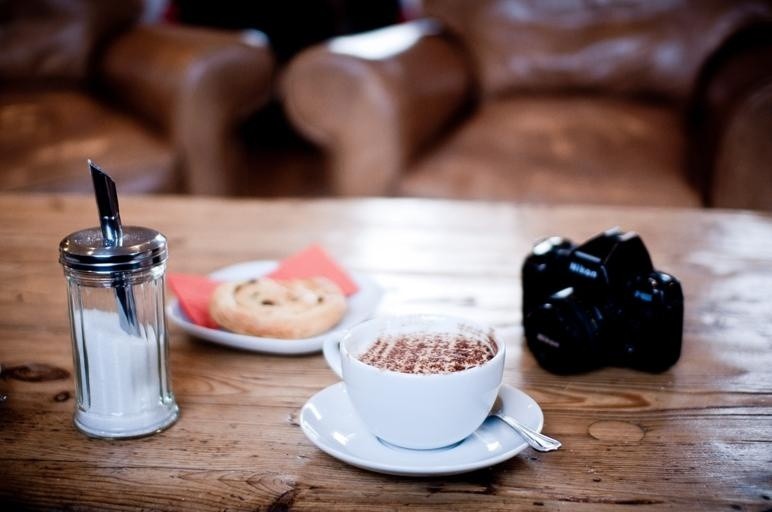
[0,2,272,199]
[277,1,770,198]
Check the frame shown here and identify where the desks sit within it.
[0,196,771,511]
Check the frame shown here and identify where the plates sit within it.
[166,258,379,358]
[298,377,546,478]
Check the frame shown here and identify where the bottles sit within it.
[56,225,181,444]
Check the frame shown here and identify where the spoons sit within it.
[488,396,565,456]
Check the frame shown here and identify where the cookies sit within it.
[209,274,348,339]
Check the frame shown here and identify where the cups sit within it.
[317,313,506,451]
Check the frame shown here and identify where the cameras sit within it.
[521,227,685,379]
